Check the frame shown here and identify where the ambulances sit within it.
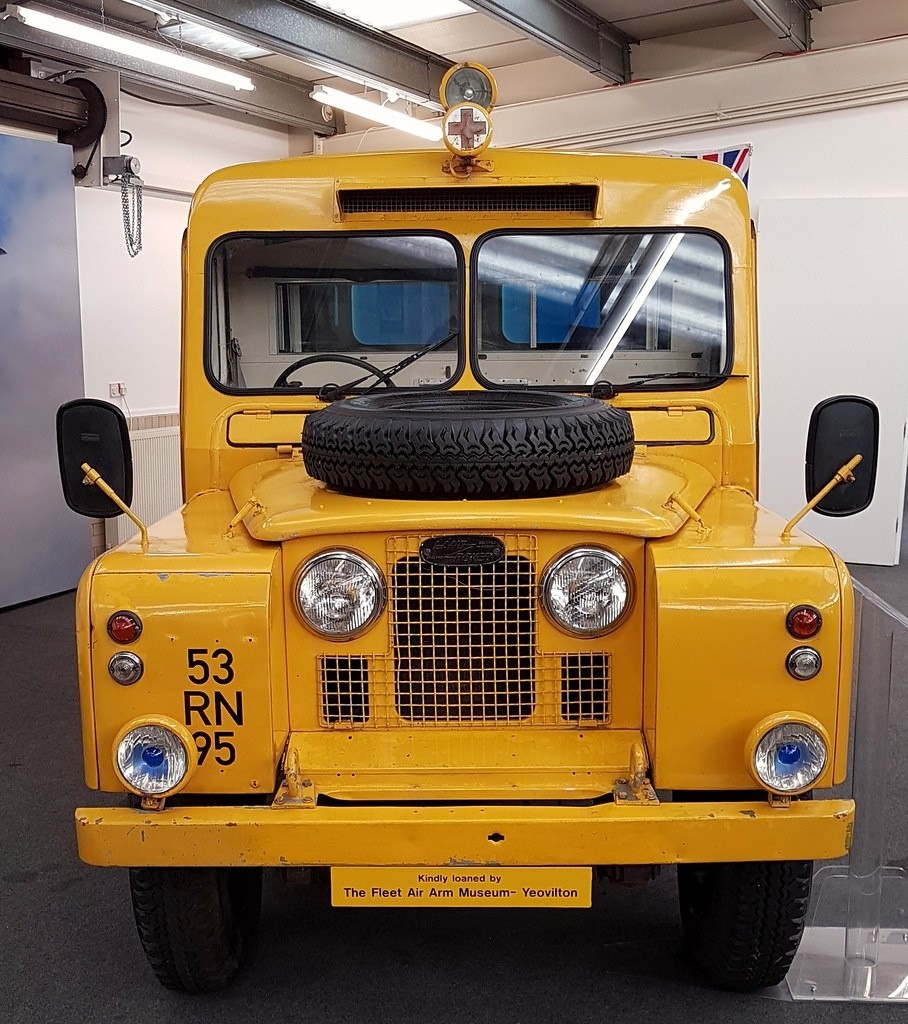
[55,58,880,996]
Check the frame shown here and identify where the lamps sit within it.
[309,84,443,142]
[0,3,257,91]
